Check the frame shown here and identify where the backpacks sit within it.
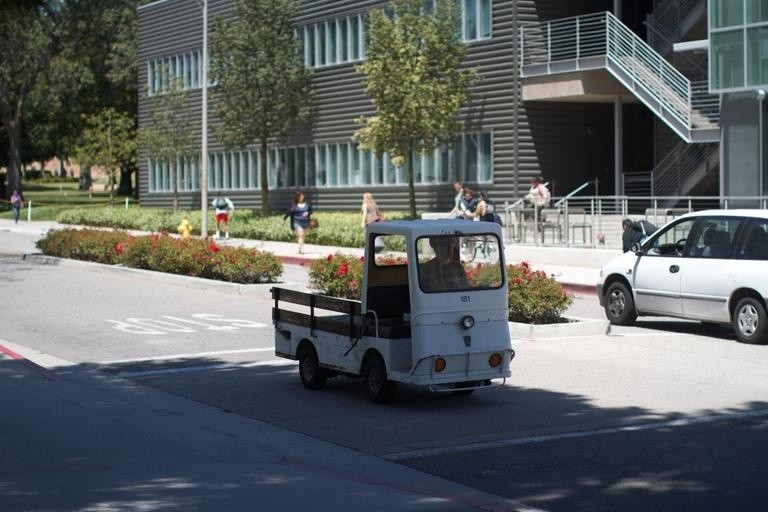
[630,219,658,236]
[215,198,227,211]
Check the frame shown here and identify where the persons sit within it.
[210,191,235,240]
[450,182,495,223]
[283,191,313,255]
[621,217,658,255]
[419,235,474,292]
[529,175,551,232]
[11,190,24,224]
[360,193,380,230]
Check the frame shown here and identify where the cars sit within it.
[597,208,767,346]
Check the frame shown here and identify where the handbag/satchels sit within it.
[377,214,384,224]
[308,218,317,228]
[493,214,502,225]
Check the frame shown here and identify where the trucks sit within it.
[270,217,515,403]
